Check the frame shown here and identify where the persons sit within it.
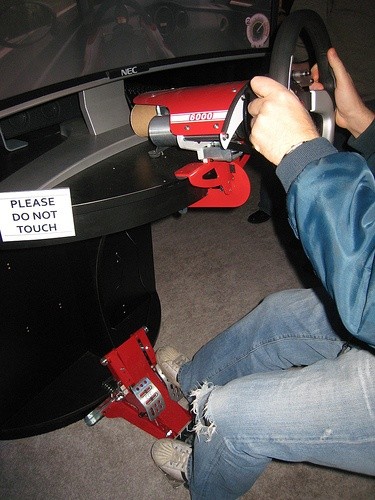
[151,47,374,500]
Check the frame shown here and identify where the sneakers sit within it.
[155,346,190,388]
[152,439,192,483]
